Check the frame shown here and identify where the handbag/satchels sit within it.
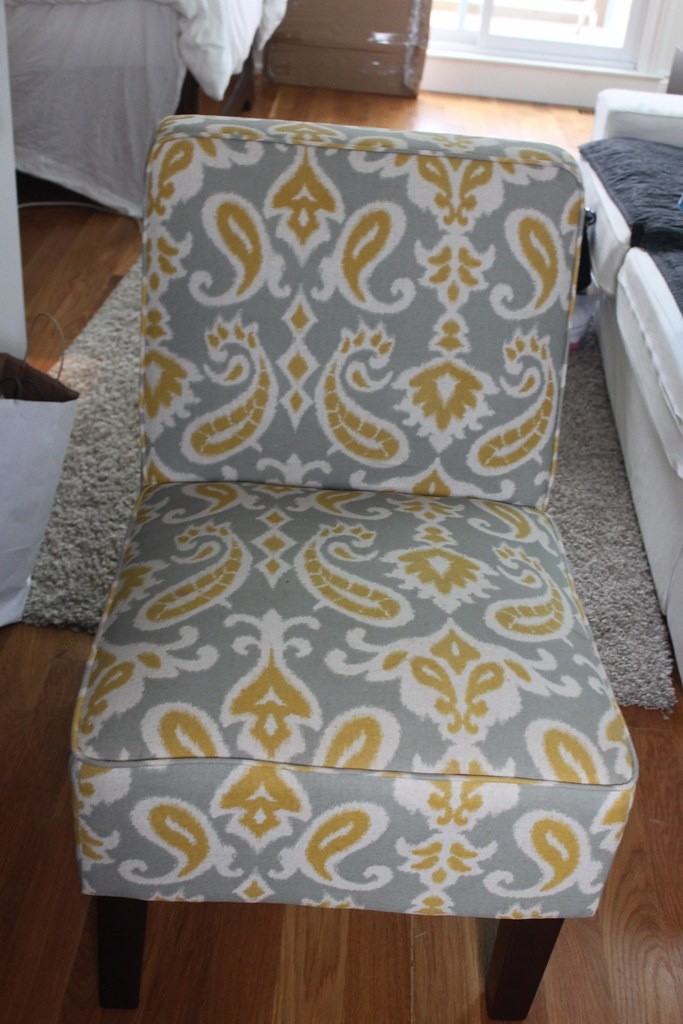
[0,312,79,628]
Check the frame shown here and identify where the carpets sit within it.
[26,246,677,708]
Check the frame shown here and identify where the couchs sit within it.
[570,90,682,672]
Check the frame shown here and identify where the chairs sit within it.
[71,113,637,1024]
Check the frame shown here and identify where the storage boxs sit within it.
[263,0,434,98]
